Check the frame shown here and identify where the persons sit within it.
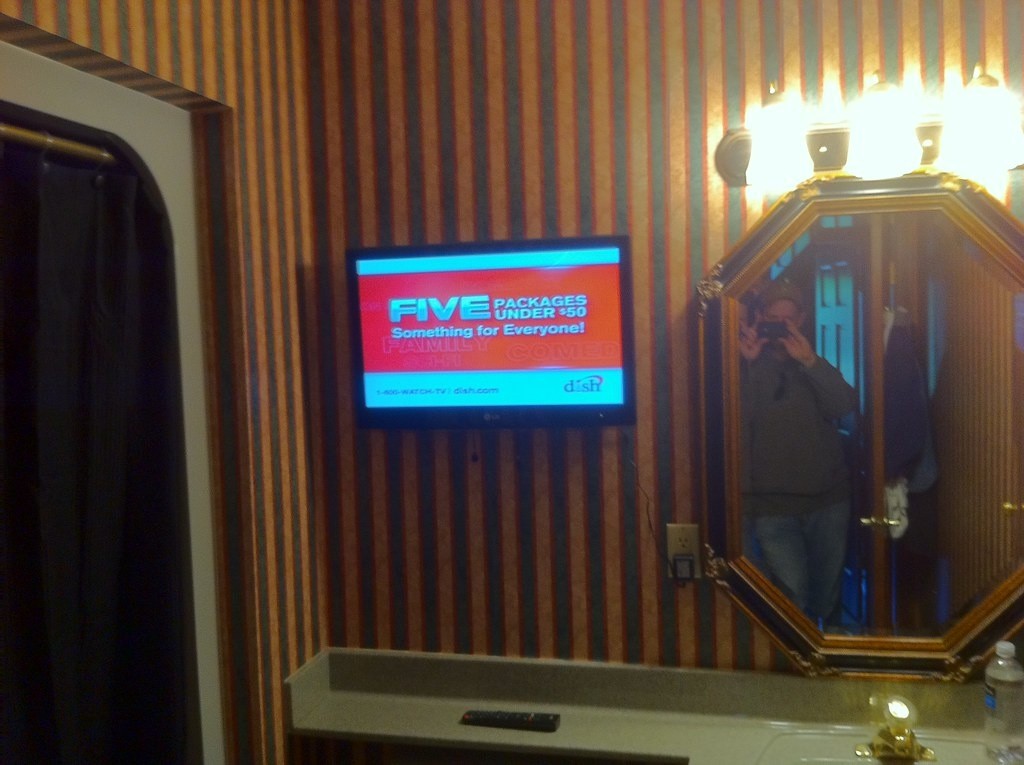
[735,268,859,637]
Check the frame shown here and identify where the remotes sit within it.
[462,710,560,733]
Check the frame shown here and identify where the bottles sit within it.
[983,640,1024,762]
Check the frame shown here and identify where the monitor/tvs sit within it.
[344,235,638,431]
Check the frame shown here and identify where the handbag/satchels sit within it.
[885,483,909,538]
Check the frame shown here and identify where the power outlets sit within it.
[665,523,702,581]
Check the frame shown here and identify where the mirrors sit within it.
[695,171,1023,683]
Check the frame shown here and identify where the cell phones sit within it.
[757,321,790,339]
